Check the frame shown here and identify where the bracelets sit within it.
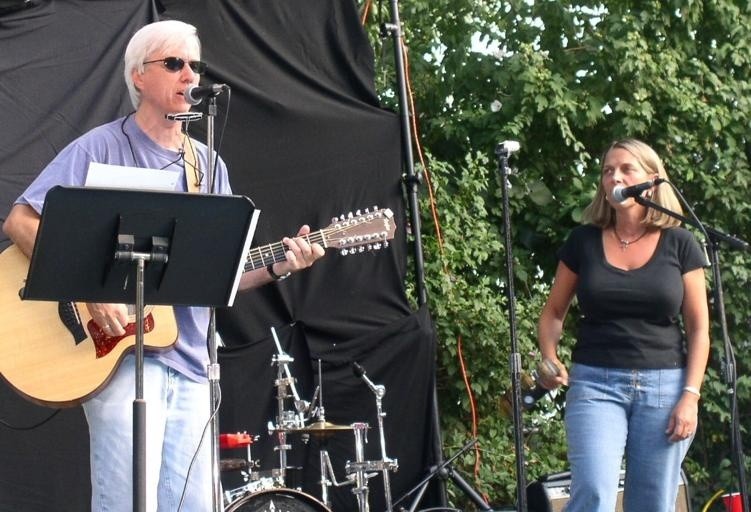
[267,264,292,280]
[683,385,700,397]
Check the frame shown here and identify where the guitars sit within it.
[0,206,397,409]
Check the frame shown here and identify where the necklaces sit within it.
[613,222,650,249]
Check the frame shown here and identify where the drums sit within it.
[224,481,332,512]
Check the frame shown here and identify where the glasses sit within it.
[142,56,206,73]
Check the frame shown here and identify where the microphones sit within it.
[184,83,223,105]
[494,140,520,153]
[611,181,654,203]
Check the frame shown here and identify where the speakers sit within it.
[527,467,691,512]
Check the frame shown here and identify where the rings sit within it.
[103,325,110,330]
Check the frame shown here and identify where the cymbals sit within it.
[294,421,373,441]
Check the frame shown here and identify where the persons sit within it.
[1,19,326,512]
[533,139,712,512]
